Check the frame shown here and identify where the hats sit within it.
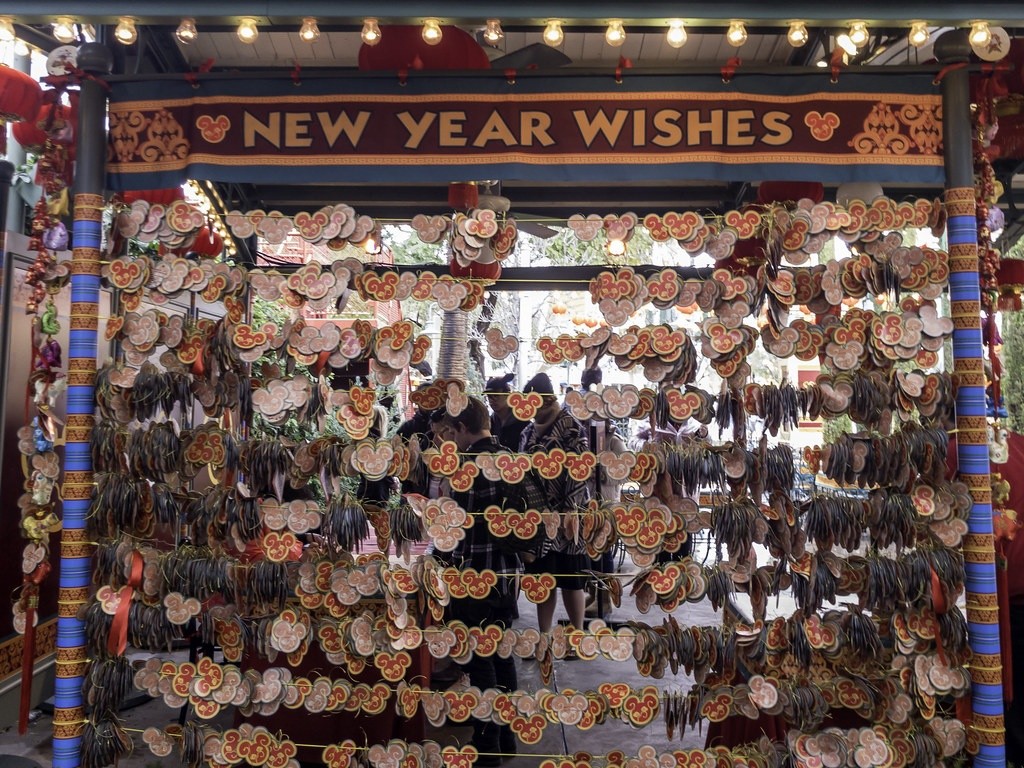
[481,374,514,396]
[523,373,553,393]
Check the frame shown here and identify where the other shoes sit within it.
[458,741,517,766]
[564,650,580,661]
[522,643,539,660]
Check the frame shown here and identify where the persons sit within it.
[396,382,434,502]
[480,373,531,453]
[561,365,602,426]
[431,396,522,768]
[407,405,463,500]
[588,423,633,511]
[622,380,711,605]
[515,373,592,638]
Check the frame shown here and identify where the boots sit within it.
[584,584,612,618]
